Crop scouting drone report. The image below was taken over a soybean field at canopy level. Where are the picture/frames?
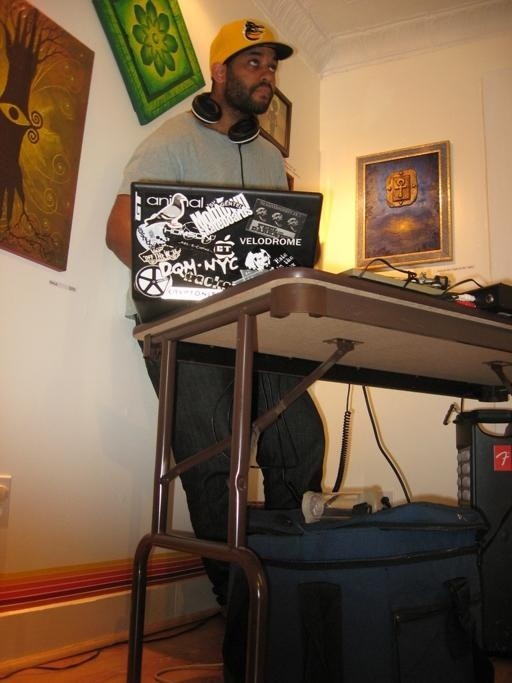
[261,84,292,157]
[354,141,453,270]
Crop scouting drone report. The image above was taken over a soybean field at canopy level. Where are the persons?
[106,18,326,605]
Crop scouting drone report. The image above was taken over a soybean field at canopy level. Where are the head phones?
[191,92,261,144]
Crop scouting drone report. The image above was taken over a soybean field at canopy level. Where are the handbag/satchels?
[221,489,494,683]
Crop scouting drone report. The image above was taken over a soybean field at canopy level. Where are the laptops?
[128,181,324,323]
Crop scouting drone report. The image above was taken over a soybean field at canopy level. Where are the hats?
[210,18,294,77]
